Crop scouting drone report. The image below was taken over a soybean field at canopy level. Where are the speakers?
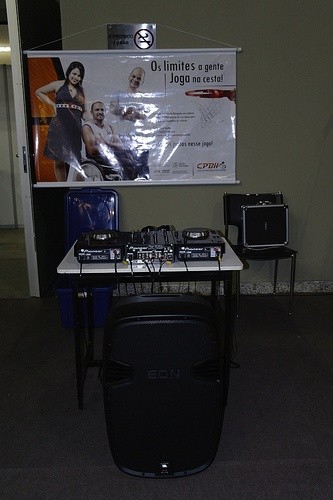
[101,293,230,479]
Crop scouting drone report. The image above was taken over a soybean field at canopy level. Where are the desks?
[57,237,243,411]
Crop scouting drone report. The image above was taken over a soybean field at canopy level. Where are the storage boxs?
[54,275,112,328]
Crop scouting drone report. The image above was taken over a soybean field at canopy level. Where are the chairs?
[223,191,299,318]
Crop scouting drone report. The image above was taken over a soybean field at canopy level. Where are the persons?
[34,61,85,182]
[108,67,151,180]
[81,101,150,181]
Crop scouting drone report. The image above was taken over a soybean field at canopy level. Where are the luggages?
[98,293,231,479]
